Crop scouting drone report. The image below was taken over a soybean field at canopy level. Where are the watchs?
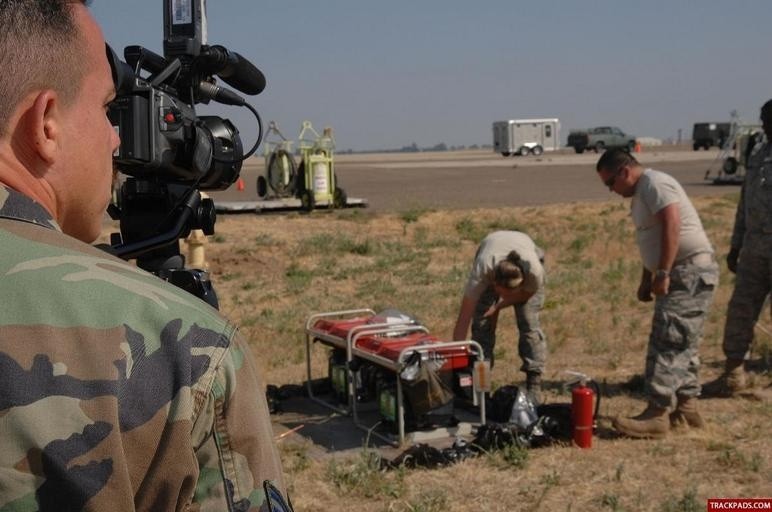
[656,269,670,279]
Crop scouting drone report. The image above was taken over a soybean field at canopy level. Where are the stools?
[302,307,486,447]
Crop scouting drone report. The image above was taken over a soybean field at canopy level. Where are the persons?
[699,99,772,396]
[0,0,292,511]
[452,230,548,409]
[595,147,719,439]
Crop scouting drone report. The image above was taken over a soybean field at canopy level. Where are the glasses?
[605,161,629,186]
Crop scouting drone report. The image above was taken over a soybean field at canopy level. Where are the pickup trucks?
[566,127,636,155]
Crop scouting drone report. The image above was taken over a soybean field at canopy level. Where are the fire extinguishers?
[567,371,601,449]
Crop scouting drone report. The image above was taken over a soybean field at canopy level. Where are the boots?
[670,395,701,431]
[613,407,671,438]
[701,359,746,399]
[526,371,542,411]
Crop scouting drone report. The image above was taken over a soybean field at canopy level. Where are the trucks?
[692,122,729,150]
